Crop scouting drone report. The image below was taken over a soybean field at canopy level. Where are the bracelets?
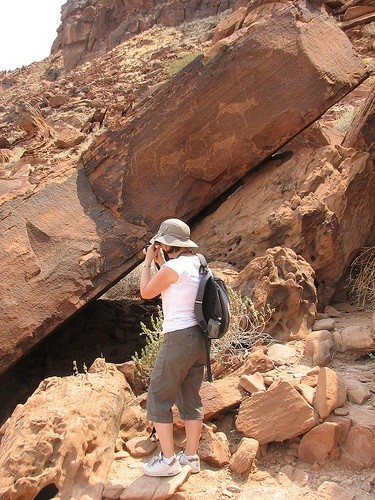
[142,264,151,268]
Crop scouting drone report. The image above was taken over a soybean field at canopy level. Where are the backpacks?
[195,254,231,339]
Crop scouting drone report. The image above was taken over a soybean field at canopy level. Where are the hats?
[149,218,199,247]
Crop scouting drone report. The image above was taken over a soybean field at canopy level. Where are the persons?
[139,218,214,475]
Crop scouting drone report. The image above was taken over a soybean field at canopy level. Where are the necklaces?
[176,251,192,258]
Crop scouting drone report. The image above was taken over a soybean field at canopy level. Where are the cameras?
[143,245,160,256]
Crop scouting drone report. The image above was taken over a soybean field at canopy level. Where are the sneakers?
[142,450,181,477]
[177,451,200,473]
